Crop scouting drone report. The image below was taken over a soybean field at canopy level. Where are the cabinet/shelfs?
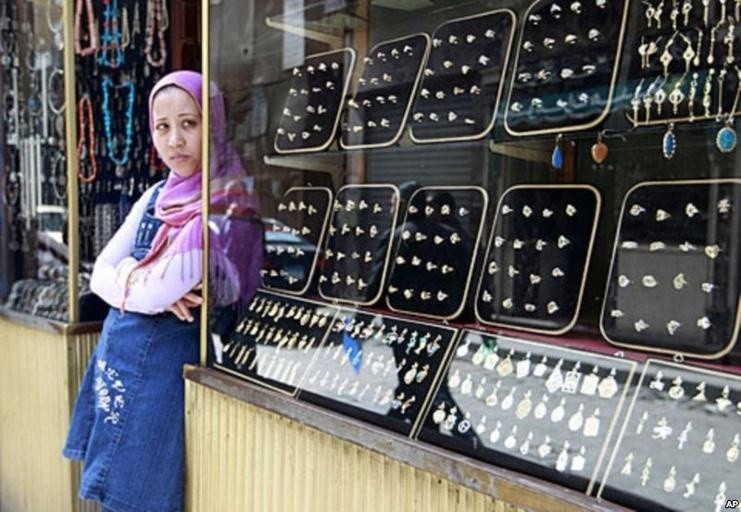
[1,2,177,512]
[183,0,738,512]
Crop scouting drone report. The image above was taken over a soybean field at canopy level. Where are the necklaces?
[225,297,739,512]
[1,0,169,324]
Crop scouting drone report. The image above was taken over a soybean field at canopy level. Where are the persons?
[65,70,264,512]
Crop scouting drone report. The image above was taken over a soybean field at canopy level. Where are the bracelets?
[630,0,740,128]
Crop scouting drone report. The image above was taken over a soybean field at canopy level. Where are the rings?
[276,0,607,141]
[261,197,732,339]
